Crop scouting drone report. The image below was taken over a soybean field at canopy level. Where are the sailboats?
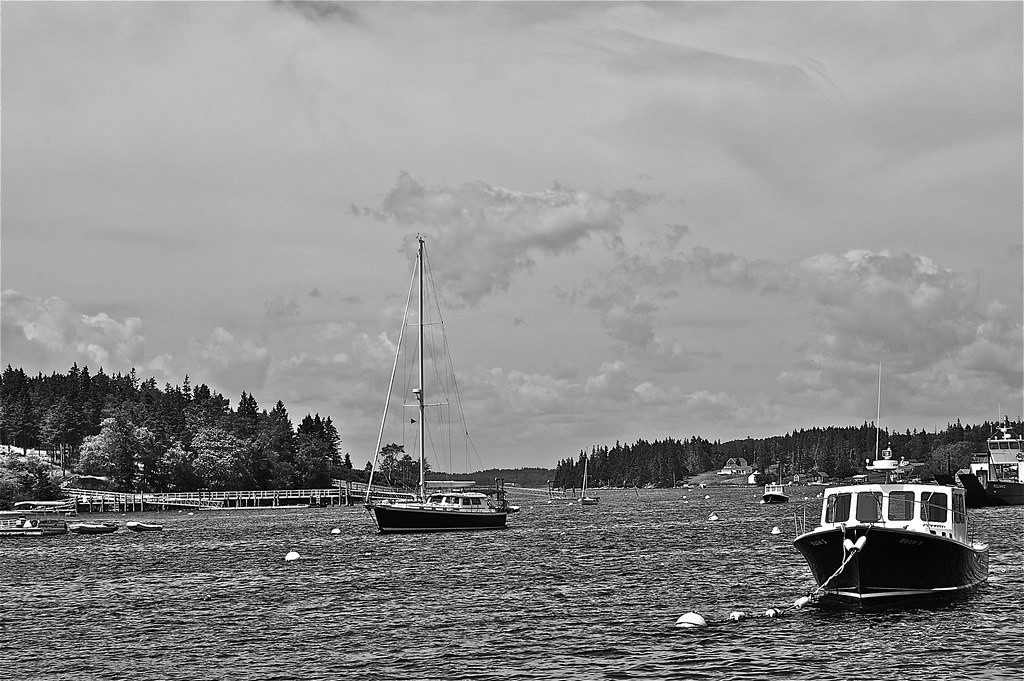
[578,458,601,506]
[362,229,510,535]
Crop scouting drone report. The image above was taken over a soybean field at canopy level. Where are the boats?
[791,358,997,609]
[952,403,1024,511]
[0,511,71,538]
[68,519,120,535]
[762,461,793,504]
[126,521,163,533]
